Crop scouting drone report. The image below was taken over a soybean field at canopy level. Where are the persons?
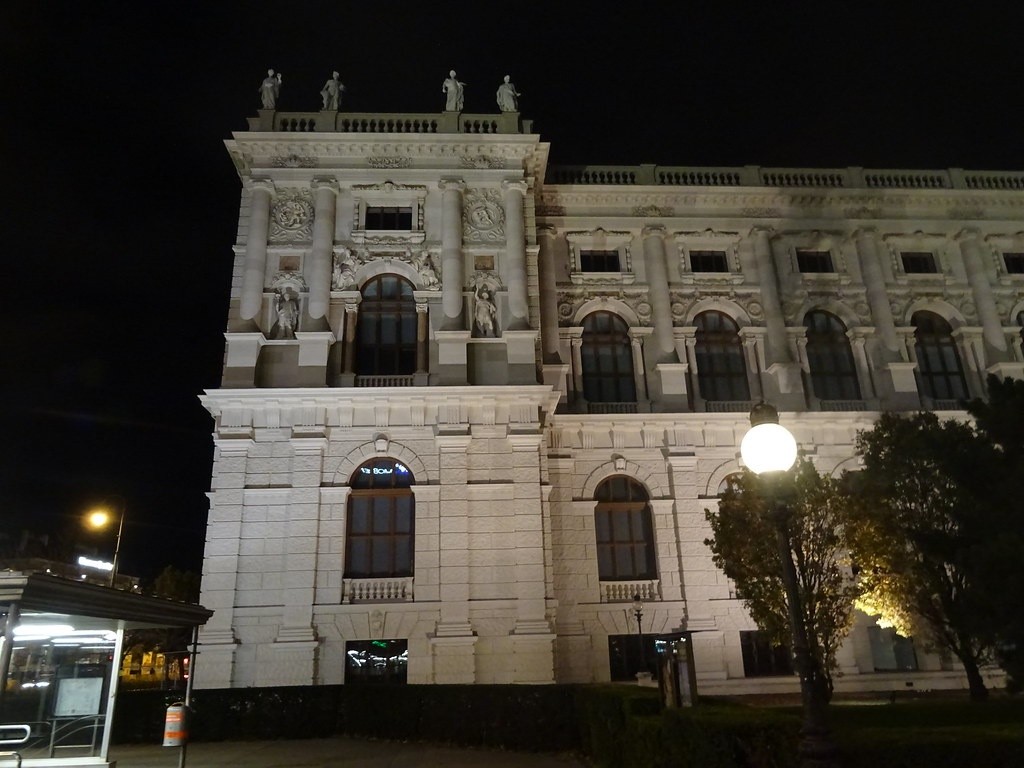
[258,70,282,109]
[497,75,521,112]
[474,284,496,338]
[275,293,300,338]
[320,71,344,110]
[443,70,466,112]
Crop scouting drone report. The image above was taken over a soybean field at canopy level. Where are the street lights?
[92,494,128,588]
[741,400,839,768]
[632,594,654,685]
